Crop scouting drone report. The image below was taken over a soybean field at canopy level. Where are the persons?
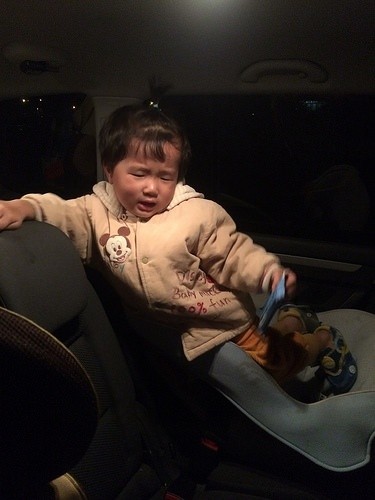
[0,98,359,394]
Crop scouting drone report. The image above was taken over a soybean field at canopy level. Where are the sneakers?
[277,303,321,332]
[314,325,357,393]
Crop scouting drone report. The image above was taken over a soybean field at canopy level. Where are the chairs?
[0,219,375,500]
